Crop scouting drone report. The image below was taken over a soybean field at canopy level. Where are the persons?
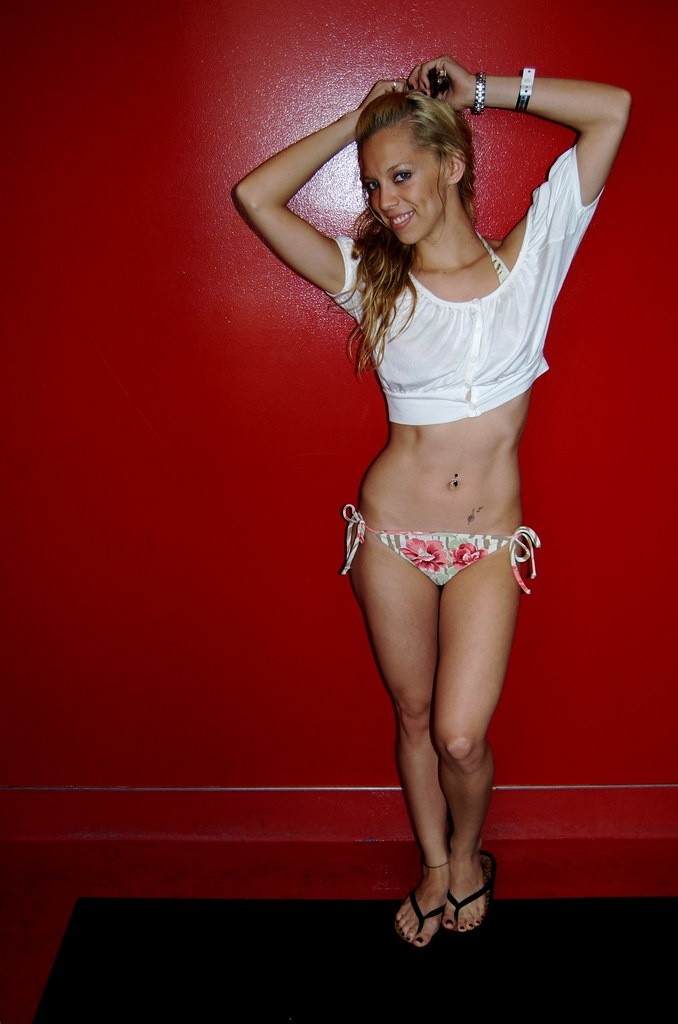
[232,52,633,950]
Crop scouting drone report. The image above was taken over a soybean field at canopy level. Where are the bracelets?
[471,71,487,117]
[513,67,536,113]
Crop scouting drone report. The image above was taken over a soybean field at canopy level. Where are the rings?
[435,70,447,78]
[392,80,399,91]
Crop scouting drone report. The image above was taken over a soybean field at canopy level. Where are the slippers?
[440,849,497,938]
[393,885,445,954]
